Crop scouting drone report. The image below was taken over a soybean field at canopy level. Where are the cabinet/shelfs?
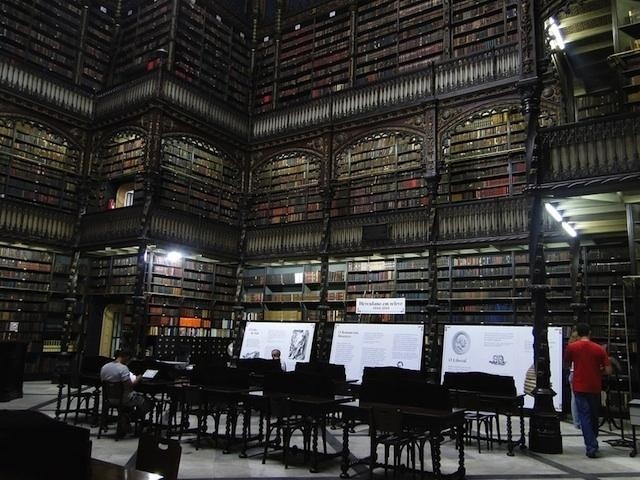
[1,1,639,344]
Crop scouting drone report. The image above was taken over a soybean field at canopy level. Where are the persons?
[289,331,307,360]
[100,348,147,435]
[271,349,288,372]
[567,328,582,429]
[563,321,613,459]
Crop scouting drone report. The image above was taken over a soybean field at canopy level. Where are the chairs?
[48,350,527,478]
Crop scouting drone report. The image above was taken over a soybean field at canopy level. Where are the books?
[587,329,640,413]
[129,372,137,383]
[1,0,639,328]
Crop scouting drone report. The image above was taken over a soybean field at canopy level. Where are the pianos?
[441,372,525,456]
[336,366,468,477]
[54,354,117,433]
[175,353,355,473]
[123,359,193,441]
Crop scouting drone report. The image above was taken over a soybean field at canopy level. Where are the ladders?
[608,284,632,407]
